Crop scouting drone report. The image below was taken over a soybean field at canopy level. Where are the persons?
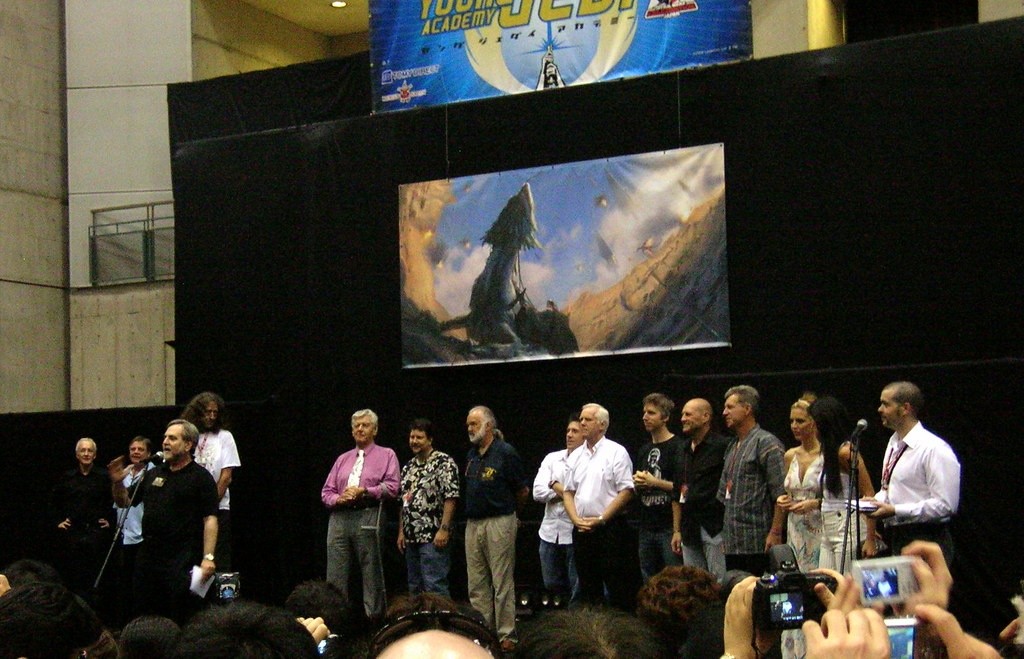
[782,394,888,574]
[0,541,1024,659]
[322,409,401,616]
[181,392,241,604]
[463,406,529,651]
[716,385,785,595]
[633,392,731,589]
[397,419,461,598]
[107,419,220,626]
[532,402,636,615]
[43,435,157,636]
[861,381,962,565]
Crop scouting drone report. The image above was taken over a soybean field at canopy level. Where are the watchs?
[599,515,607,525]
[440,523,450,531]
[549,479,559,489]
[203,553,215,561]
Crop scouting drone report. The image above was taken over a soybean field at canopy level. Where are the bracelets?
[817,497,823,507]
[363,486,368,494]
[673,529,681,533]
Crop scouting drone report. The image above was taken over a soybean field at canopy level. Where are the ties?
[882,445,906,505]
[347,450,365,489]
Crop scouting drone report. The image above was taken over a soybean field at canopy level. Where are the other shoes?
[500,640,514,652]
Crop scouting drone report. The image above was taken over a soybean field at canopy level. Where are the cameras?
[881,614,940,659]
[317,634,339,655]
[751,560,838,632]
[848,556,922,605]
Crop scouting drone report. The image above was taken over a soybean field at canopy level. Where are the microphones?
[139,450,164,463]
[850,419,869,440]
